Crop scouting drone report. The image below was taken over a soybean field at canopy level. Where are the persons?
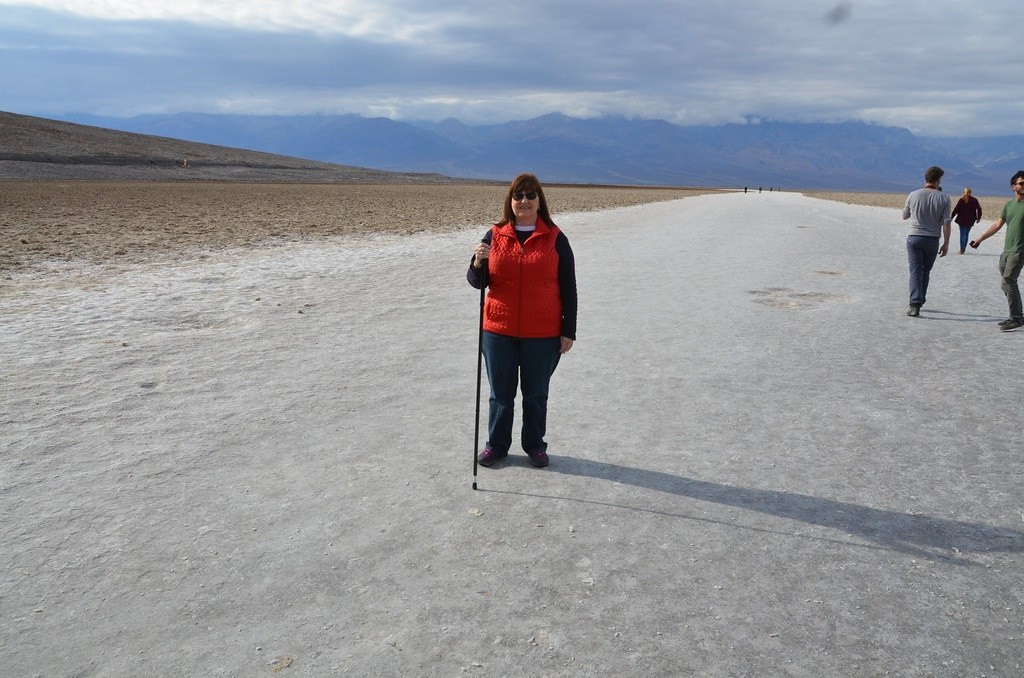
[902,166,951,315]
[466,173,578,467]
[970,171,1024,331]
[951,189,982,254]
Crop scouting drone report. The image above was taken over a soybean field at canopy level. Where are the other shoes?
[906,306,919,316]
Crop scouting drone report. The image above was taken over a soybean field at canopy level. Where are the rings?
[481,251,483,254]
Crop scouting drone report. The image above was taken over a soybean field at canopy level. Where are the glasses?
[511,192,537,200]
[1015,182,1024,186]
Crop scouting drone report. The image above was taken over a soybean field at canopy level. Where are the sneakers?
[477,447,509,466]
[521,444,550,467]
[999,319,1023,331]
[999,316,1024,325]
